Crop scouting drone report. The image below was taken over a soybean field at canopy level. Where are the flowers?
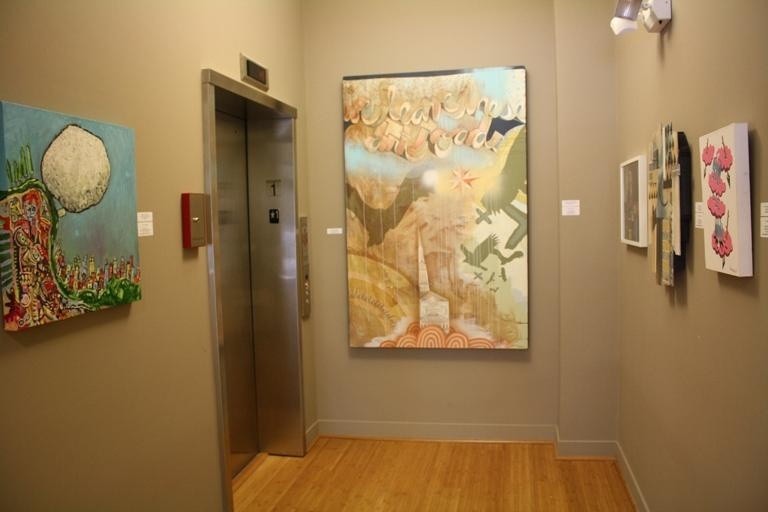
[702,136,733,269]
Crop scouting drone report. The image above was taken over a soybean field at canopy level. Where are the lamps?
[610,0,672,35]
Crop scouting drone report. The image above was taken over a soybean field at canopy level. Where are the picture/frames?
[620,155,648,247]
